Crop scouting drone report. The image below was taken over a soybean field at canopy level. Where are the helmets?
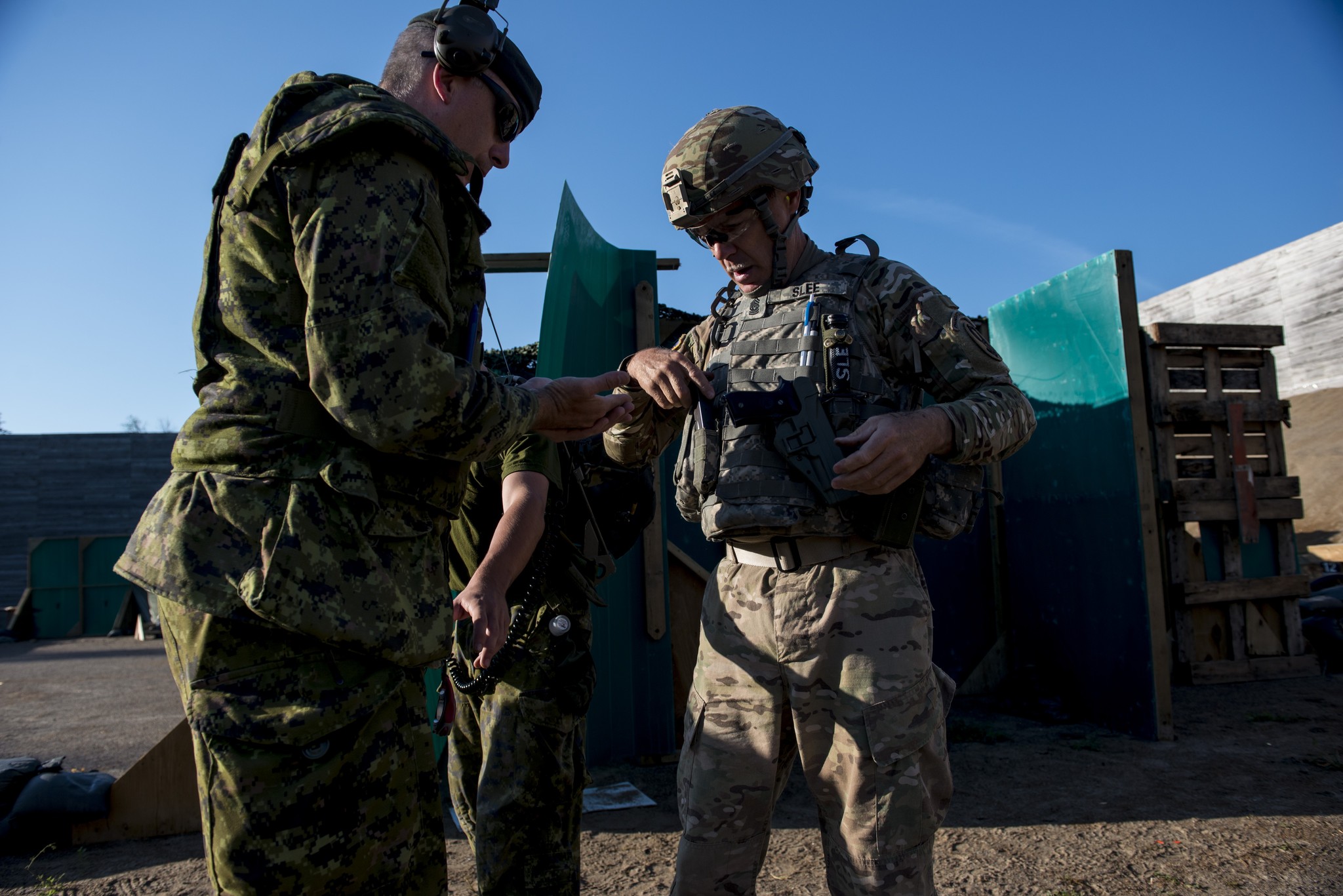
[659,105,819,231]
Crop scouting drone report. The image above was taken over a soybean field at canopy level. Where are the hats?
[406,7,543,135]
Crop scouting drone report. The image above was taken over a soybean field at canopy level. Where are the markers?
[806,302,821,365]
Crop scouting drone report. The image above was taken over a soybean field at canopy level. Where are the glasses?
[420,51,521,143]
[685,201,760,249]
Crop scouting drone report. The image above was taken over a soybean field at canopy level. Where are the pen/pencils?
[800,294,814,366]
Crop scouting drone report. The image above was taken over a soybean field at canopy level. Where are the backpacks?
[557,433,657,562]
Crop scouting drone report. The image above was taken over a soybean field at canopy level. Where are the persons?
[113,1,633,896]
[443,339,600,896]
[602,104,1039,896]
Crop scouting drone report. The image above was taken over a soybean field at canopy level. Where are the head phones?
[433,2,508,77]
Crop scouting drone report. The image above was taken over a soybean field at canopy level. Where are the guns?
[720,375,857,506]
